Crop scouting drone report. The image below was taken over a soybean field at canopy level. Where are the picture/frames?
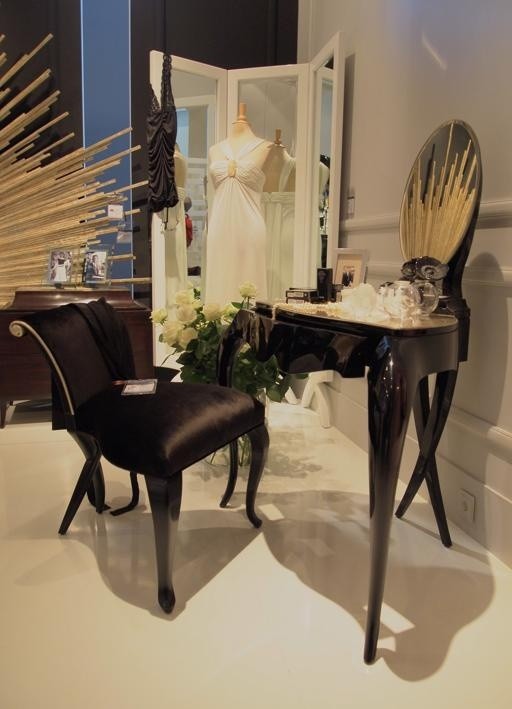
[0,287,155,429]
[217,300,472,667]
[332,248,368,290]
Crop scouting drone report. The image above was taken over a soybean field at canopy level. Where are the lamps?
[8,296,271,615]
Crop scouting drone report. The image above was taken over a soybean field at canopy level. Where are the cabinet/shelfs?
[375,286,390,314]
[383,281,421,320]
[407,278,439,317]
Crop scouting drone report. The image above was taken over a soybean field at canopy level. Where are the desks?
[217,300,472,667]
[0,287,155,429]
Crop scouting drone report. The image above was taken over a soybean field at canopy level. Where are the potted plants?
[332,248,368,290]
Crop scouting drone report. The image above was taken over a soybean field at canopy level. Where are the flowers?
[148,281,308,467]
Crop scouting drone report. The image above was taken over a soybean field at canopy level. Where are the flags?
[204,418,272,480]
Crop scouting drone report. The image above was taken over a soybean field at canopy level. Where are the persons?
[50,251,71,284]
[342,267,353,288]
[165,103,331,342]
[87,255,104,280]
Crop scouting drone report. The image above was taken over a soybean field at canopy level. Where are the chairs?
[8,296,271,615]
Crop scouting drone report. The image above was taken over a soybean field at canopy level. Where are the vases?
[148,281,308,467]
[204,418,272,480]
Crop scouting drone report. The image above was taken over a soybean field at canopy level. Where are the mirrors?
[148,50,226,310]
[309,32,345,290]
[398,119,484,266]
[227,63,309,299]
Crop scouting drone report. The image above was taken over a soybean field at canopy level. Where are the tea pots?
[398,119,484,266]
